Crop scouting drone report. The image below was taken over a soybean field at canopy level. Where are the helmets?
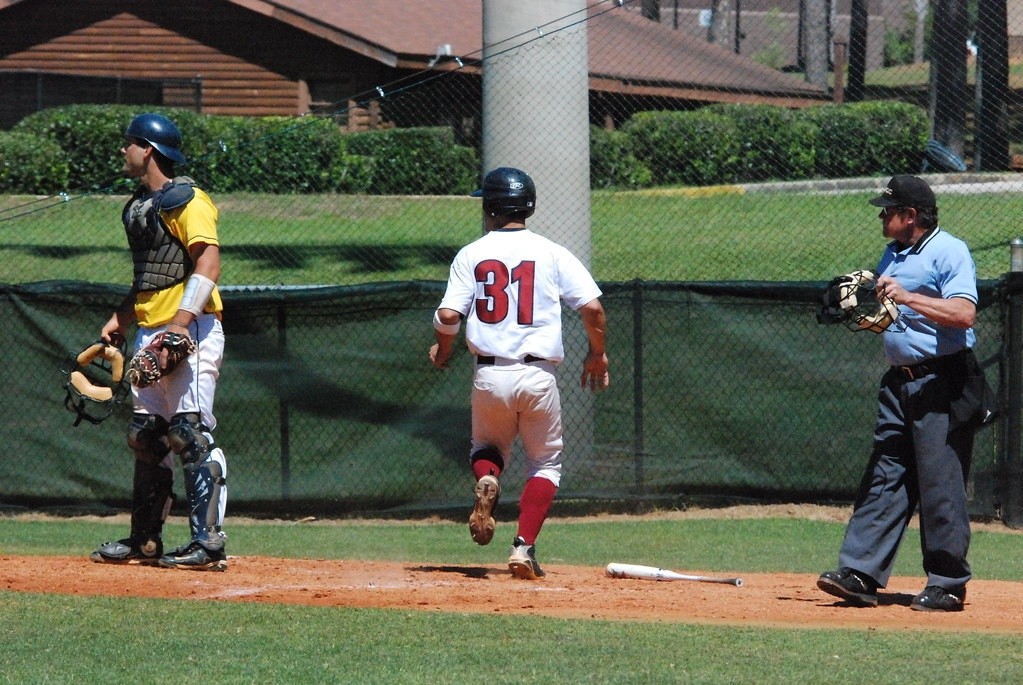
[471,168,536,217]
[126,113,186,162]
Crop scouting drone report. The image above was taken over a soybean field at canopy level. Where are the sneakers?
[911,586,967,612]
[159,541,227,570]
[469,468,501,545]
[90,537,163,565]
[818,568,878,606]
[510,536,546,579]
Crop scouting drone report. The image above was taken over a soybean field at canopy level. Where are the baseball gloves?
[129,331,196,388]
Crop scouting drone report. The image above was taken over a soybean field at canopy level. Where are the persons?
[97,113,228,566]
[817,176,978,610]
[428,166,609,580]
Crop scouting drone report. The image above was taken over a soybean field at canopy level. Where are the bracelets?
[432,311,461,335]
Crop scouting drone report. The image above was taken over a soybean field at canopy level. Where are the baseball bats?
[605,562,743,587]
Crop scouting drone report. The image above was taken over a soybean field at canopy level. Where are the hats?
[869,175,935,207]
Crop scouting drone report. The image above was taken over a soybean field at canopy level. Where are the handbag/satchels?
[935,350,1000,444]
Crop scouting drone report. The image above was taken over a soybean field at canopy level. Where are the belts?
[478,354,546,364]
[894,357,941,381]
